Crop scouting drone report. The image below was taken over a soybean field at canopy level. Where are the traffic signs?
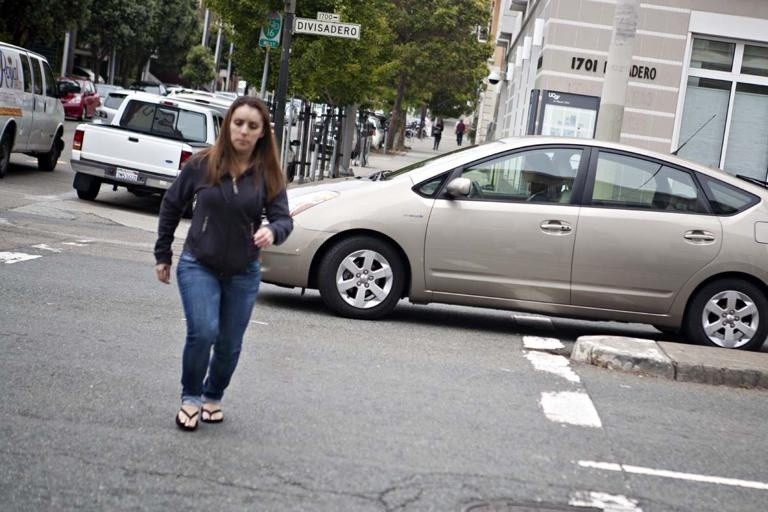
[293,10,362,41]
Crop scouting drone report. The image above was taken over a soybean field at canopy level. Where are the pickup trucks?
[71,92,223,219]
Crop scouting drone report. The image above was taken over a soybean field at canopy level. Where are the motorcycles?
[406,121,426,138]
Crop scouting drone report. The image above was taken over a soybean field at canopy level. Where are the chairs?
[556,171,576,202]
[650,184,670,209]
[666,188,695,211]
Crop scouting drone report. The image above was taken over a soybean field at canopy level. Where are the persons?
[432,116,443,150]
[456,119,465,146]
[521,153,560,201]
[154,96,293,430]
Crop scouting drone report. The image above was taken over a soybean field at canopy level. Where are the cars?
[259,134,764,350]
[89,88,144,127]
[56,75,101,121]
[146,81,386,178]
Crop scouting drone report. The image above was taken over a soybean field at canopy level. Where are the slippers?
[176,406,200,432]
[201,407,223,424]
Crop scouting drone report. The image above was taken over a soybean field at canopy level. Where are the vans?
[0,40,68,178]
